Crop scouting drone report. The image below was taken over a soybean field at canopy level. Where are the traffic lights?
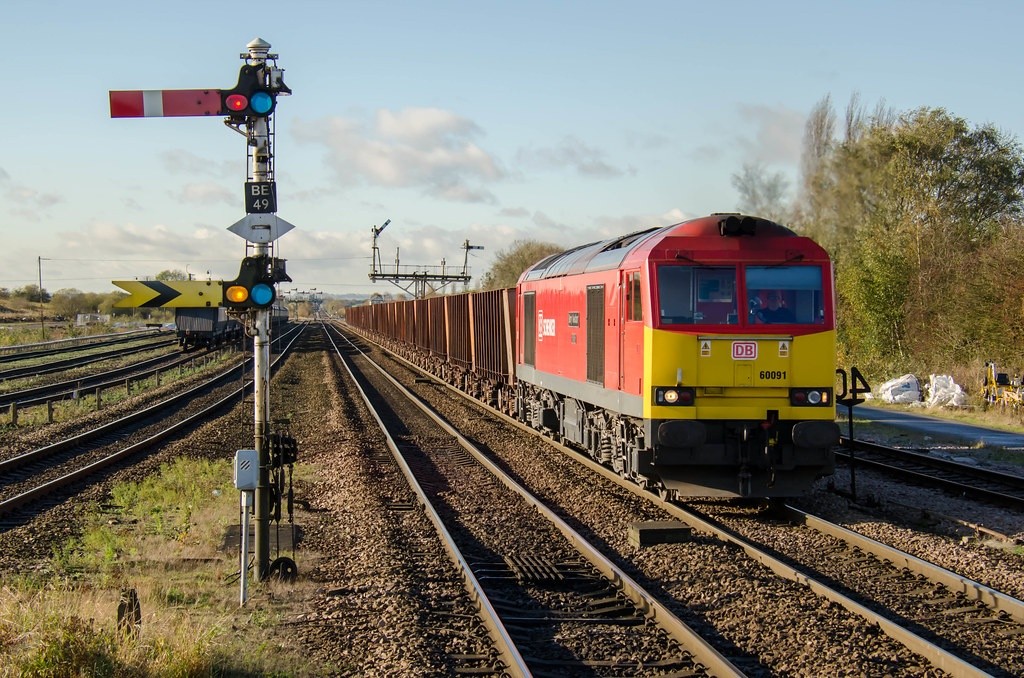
[218,94,277,115]
[225,280,276,308]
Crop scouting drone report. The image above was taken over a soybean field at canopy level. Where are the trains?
[342,215,845,498]
